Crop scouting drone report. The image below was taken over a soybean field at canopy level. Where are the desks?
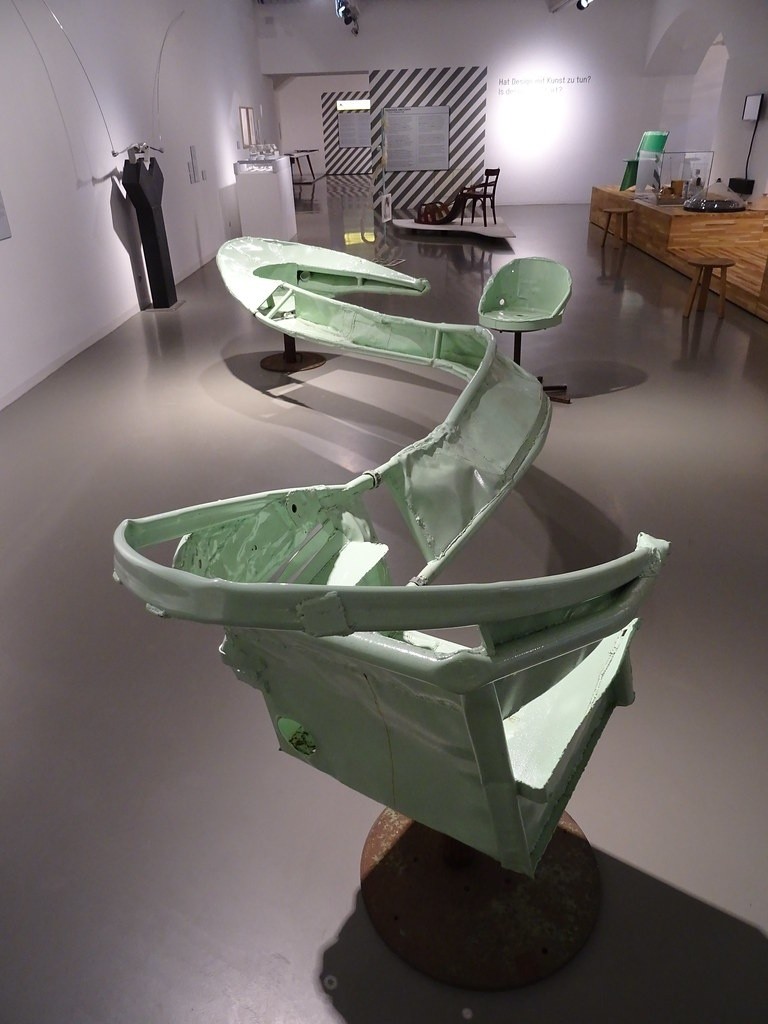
[682,256,736,319]
[287,147,319,180]
[601,207,633,251]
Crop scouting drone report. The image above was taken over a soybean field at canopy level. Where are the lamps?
[335,0,359,38]
[336,99,370,110]
[729,91,765,194]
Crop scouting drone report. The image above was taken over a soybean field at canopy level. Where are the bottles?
[691,168,703,186]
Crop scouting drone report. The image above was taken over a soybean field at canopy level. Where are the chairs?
[417,167,503,228]
[125,232,664,976]
